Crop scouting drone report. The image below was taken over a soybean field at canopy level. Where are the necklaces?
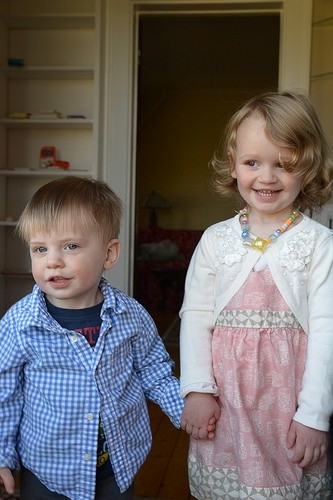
[238,204,301,251]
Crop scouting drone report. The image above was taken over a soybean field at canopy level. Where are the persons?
[176,92,333,500]
[1,174,216,500]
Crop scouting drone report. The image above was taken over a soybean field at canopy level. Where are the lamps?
[138,190,175,226]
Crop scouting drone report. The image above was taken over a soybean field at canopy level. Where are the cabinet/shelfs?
[0,0,105,318]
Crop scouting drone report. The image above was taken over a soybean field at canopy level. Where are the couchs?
[134,228,207,314]
